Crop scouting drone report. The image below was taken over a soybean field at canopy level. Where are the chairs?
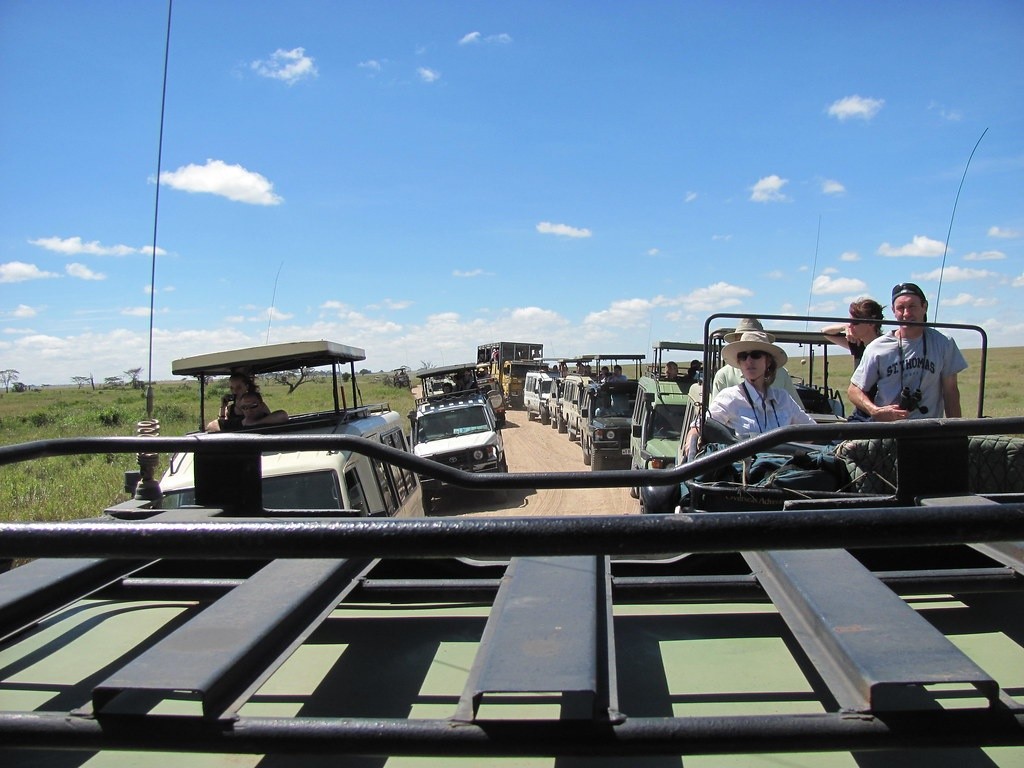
[427,416,445,438]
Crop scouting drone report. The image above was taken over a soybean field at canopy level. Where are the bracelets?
[218,415,226,418]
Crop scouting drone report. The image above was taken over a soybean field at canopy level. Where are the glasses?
[852,320,870,325]
[240,402,261,410]
[737,350,766,361]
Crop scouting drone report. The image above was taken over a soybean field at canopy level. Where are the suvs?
[407,362,508,514]
[630,339,848,507]
[392,368,413,392]
[675,312,1023,509]
[417,342,648,471]
[157,338,427,519]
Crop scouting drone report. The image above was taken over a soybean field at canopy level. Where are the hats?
[892,283,927,323]
[724,318,775,343]
[721,331,788,369]
[691,360,702,368]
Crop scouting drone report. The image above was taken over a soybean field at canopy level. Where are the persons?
[442,367,491,393]
[491,348,541,361]
[681,332,818,460]
[400,369,405,375]
[819,300,886,422]
[207,393,289,430]
[576,362,591,376]
[848,282,968,422]
[217,374,260,419]
[552,362,572,378]
[664,360,704,382]
[599,365,626,383]
[712,318,806,413]
